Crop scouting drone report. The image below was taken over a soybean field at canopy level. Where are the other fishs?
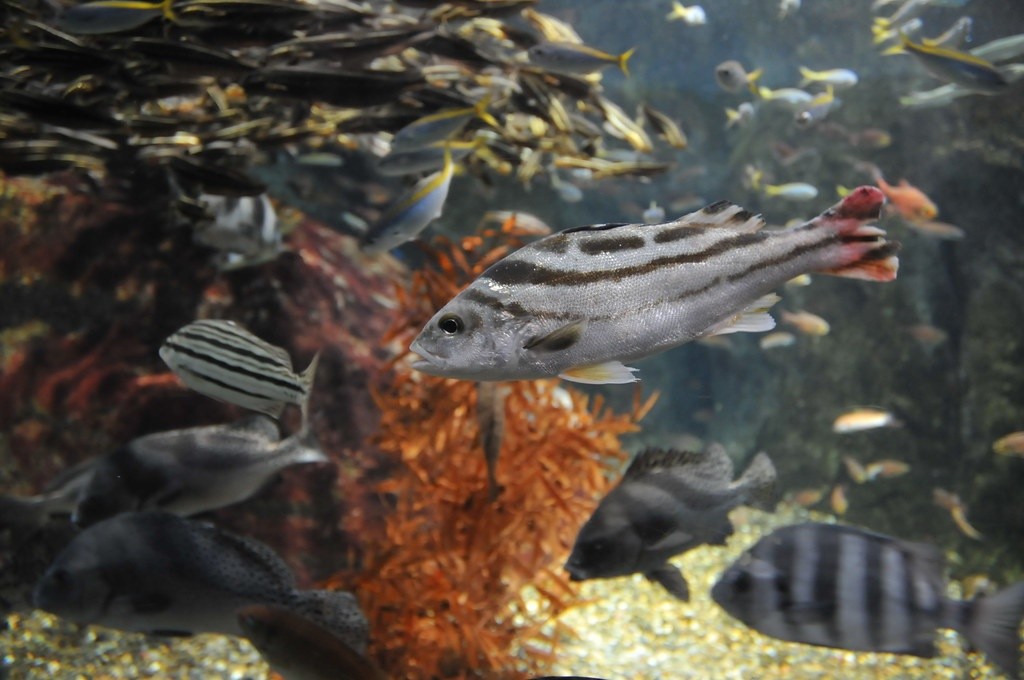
[0,0,1024,680]
